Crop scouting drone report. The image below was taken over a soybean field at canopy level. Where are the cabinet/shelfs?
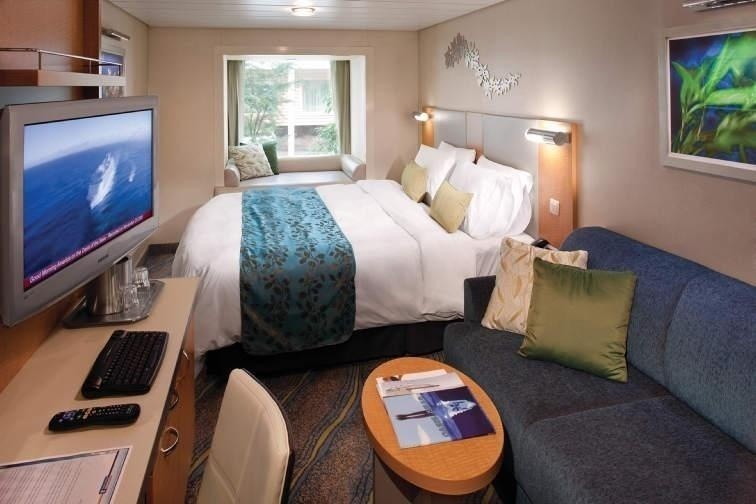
[139,298,196,503]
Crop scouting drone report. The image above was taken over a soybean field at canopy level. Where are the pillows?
[227,140,279,182]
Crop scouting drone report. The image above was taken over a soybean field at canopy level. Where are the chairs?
[197,365,296,503]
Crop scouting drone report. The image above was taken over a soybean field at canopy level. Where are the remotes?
[49,403,140,430]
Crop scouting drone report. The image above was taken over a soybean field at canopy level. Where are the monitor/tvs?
[0,95,163,328]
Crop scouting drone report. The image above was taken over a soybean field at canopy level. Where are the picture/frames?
[656,12,756,184]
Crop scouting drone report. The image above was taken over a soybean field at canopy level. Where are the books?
[374,368,497,449]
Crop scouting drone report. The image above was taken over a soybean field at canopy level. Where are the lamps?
[523,127,570,146]
[410,110,434,123]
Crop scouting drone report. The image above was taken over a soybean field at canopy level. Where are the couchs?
[440,225,756,503]
[213,152,366,195]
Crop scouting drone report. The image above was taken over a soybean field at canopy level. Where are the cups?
[84,255,137,316]
[131,266,150,290]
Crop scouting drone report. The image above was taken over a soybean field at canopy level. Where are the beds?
[183,104,579,379]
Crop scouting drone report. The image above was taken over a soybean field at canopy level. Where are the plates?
[63,278,167,328]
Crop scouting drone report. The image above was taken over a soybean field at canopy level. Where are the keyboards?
[81,329,169,400]
